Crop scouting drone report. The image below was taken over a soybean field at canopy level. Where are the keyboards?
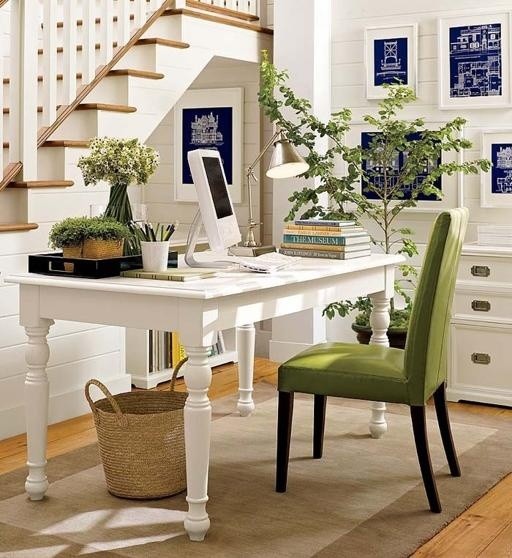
[240,256,297,273]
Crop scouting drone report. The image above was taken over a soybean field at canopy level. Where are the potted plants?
[251,46,493,350]
[49,216,127,261]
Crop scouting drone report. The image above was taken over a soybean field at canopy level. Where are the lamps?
[230,121,311,257]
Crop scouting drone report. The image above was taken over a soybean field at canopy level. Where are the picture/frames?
[172,84,247,208]
[335,8,512,219]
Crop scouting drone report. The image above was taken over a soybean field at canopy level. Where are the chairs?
[272,206,464,510]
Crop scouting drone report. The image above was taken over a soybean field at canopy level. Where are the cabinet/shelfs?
[123,237,239,389]
[444,241,511,409]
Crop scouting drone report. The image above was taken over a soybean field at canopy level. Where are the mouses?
[259,252,286,258]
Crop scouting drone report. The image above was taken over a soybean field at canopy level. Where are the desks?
[1,248,404,541]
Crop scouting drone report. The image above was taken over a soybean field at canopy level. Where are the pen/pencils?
[128,220,179,241]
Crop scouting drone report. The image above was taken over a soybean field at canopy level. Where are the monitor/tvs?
[184,149,242,269]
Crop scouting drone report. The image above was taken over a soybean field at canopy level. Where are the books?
[278,219,371,260]
[123,268,219,281]
[149,329,226,373]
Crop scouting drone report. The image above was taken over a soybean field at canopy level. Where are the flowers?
[76,134,160,223]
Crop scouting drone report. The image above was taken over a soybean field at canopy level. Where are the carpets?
[0,381,511,558]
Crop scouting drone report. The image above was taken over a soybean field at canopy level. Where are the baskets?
[62,236,125,259]
[85,355,189,500]
